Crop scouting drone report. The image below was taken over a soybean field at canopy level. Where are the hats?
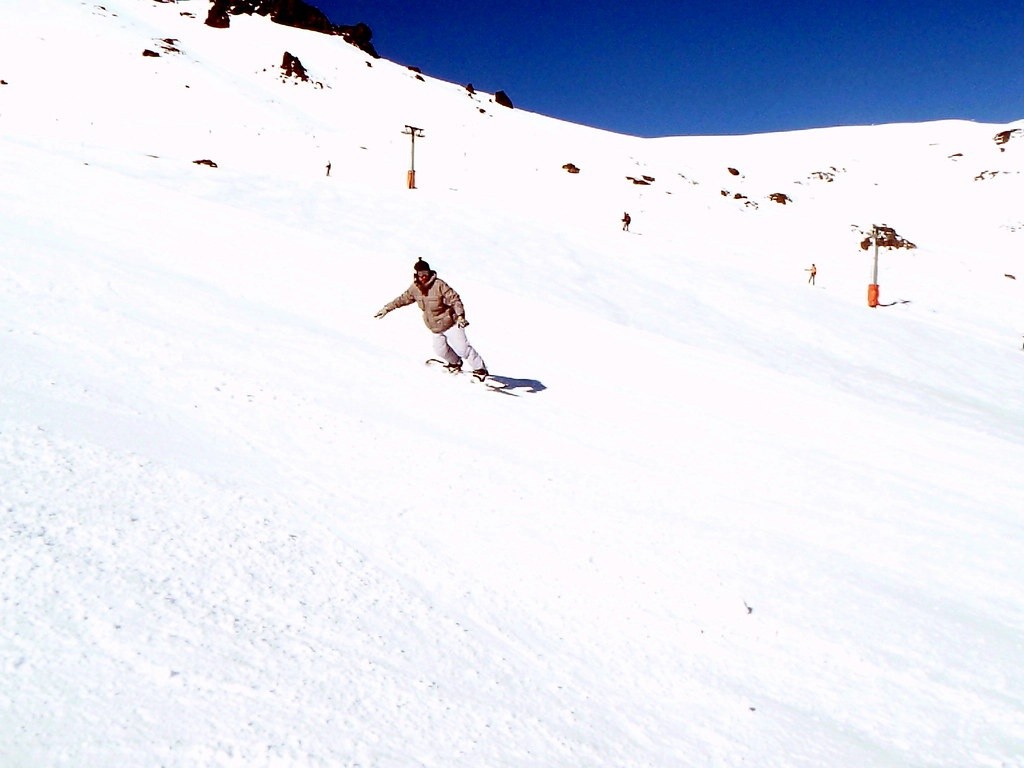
[415,257,430,271]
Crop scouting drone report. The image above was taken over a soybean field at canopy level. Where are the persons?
[326,160,332,176]
[622,214,631,231]
[374,260,489,383]
[809,264,816,285]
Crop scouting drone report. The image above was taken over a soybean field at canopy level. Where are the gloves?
[457,316,469,328]
[374,307,388,319]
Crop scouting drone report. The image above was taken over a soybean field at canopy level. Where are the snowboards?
[426,358,509,391]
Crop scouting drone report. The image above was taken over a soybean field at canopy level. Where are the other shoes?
[443,360,463,371]
[473,369,489,375]
